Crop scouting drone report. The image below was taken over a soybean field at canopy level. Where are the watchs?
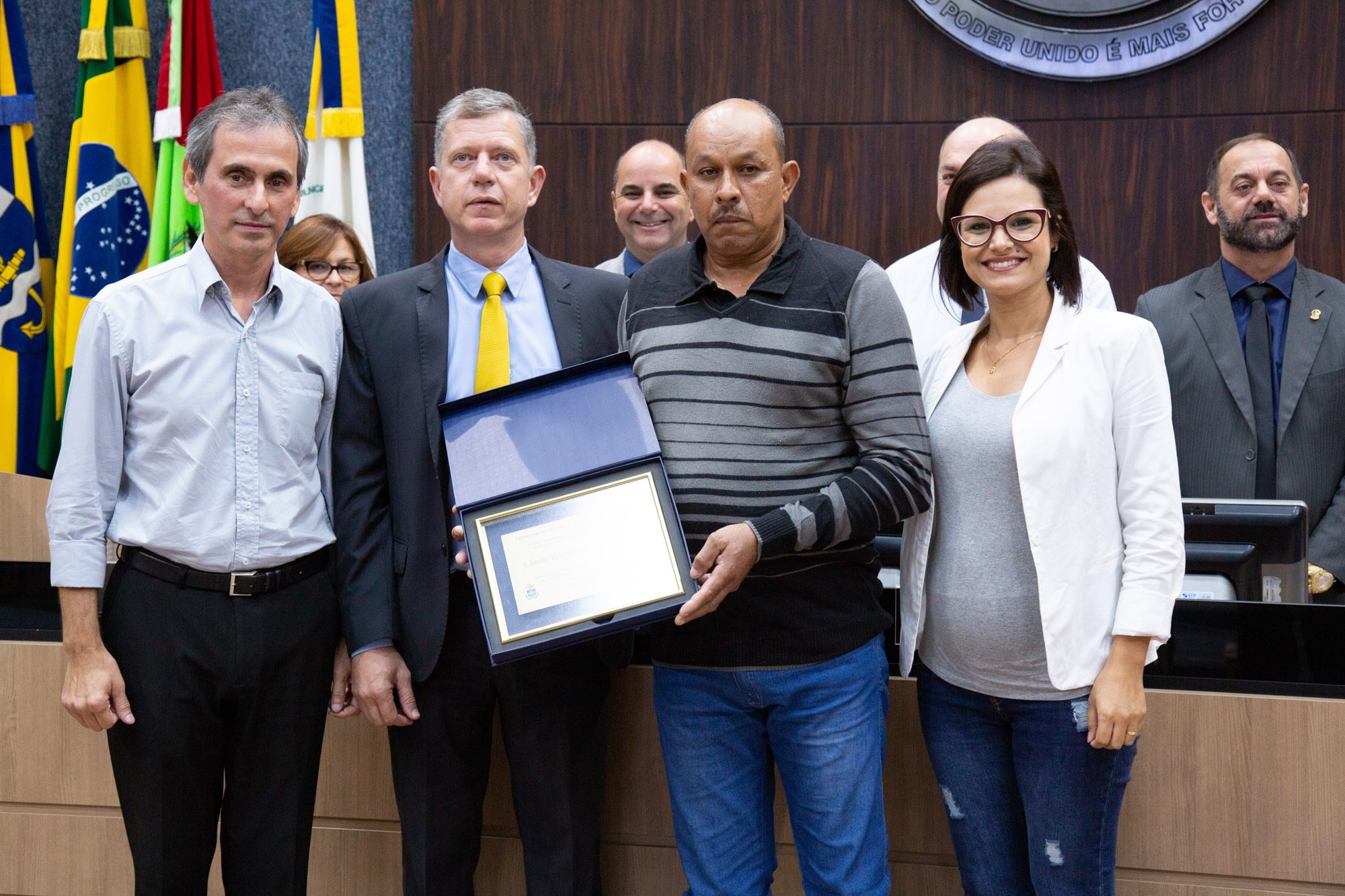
[1307,562,1335,595]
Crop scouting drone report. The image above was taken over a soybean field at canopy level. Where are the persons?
[330,87,632,896]
[883,116,1118,415]
[452,98,932,896]
[594,139,697,276]
[45,81,362,896]
[277,212,377,316]
[899,139,1187,896]
[1132,133,1344,610]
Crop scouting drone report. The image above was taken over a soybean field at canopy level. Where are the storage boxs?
[441,355,699,667]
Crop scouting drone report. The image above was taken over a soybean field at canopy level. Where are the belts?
[121,546,336,596]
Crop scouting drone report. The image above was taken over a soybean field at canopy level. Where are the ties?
[1242,284,1280,499]
[960,287,985,324]
[473,272,510,394]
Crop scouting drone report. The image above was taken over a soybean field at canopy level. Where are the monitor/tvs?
[1177,497,1308,604]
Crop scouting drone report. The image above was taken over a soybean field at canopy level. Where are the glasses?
[950,208,1052,247]
[294,259,364,282]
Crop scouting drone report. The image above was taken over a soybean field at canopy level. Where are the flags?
[0,1,365,479]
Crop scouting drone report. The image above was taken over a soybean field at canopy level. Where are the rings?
[1126,731,1137,737]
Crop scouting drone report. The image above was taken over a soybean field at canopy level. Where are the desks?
[2,601,1345,894]
